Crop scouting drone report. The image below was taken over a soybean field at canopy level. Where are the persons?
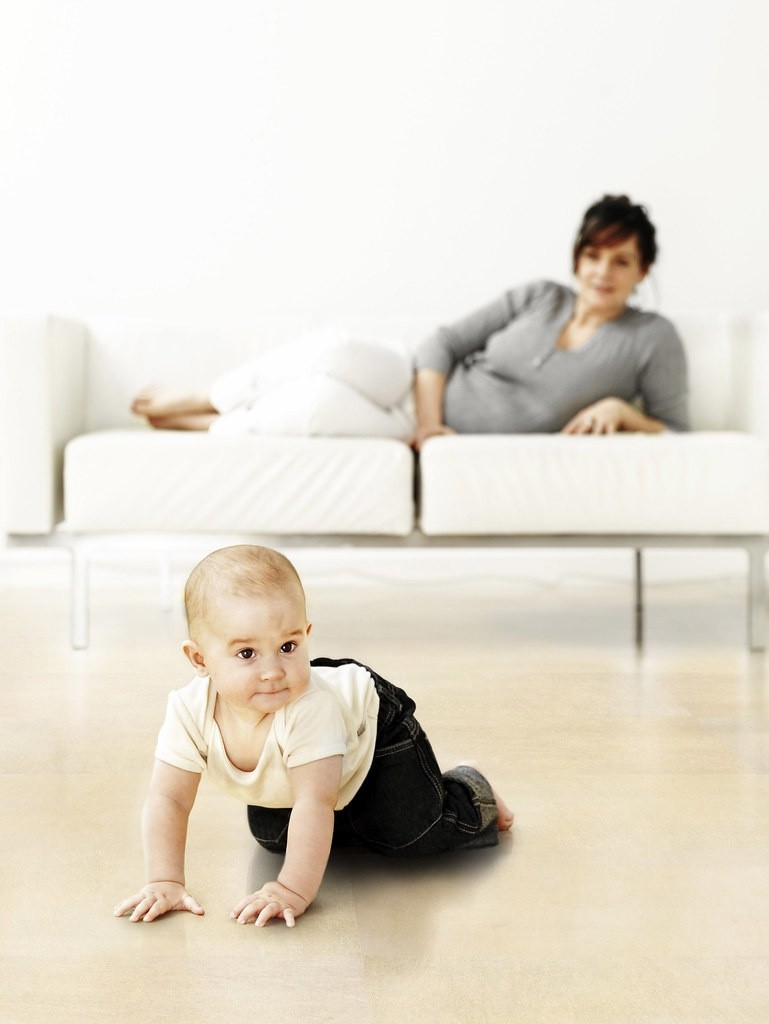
[127,196,691,441]
[113,544,514,929]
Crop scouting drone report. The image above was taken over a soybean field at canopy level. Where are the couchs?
[0,309,769,654]
